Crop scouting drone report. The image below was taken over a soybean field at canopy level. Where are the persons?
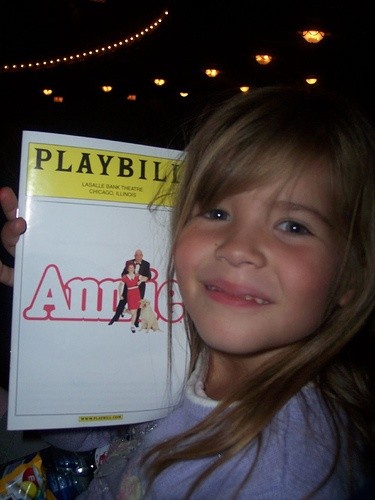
[118,261,148,332]
[108,249,151,328]
[0,83,375,500]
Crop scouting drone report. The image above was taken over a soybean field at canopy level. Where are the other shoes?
[131,327,135,334]
[134,322,139,328]
[108,318,119,326]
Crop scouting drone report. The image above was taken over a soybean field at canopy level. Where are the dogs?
[137,299,163,333]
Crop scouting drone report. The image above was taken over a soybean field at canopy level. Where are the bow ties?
[135,262,142,267]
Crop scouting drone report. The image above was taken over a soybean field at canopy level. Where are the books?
[6,131,190,431]
